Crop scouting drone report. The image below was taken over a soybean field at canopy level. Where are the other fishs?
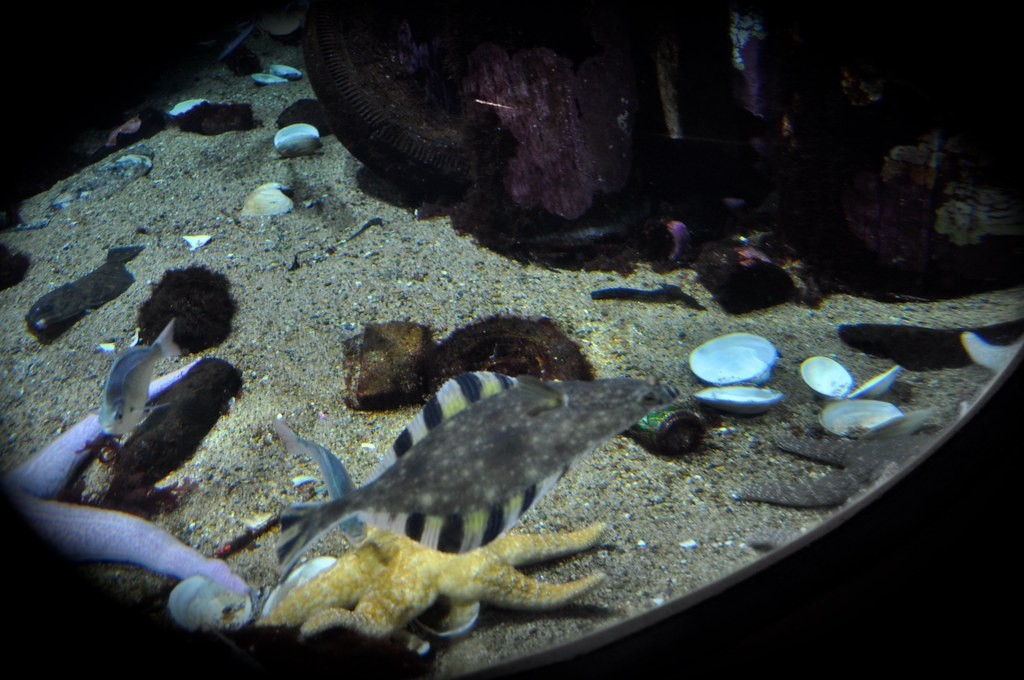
[25,246,146,346]
[96,317,181,435]
[255,371,680,588]
